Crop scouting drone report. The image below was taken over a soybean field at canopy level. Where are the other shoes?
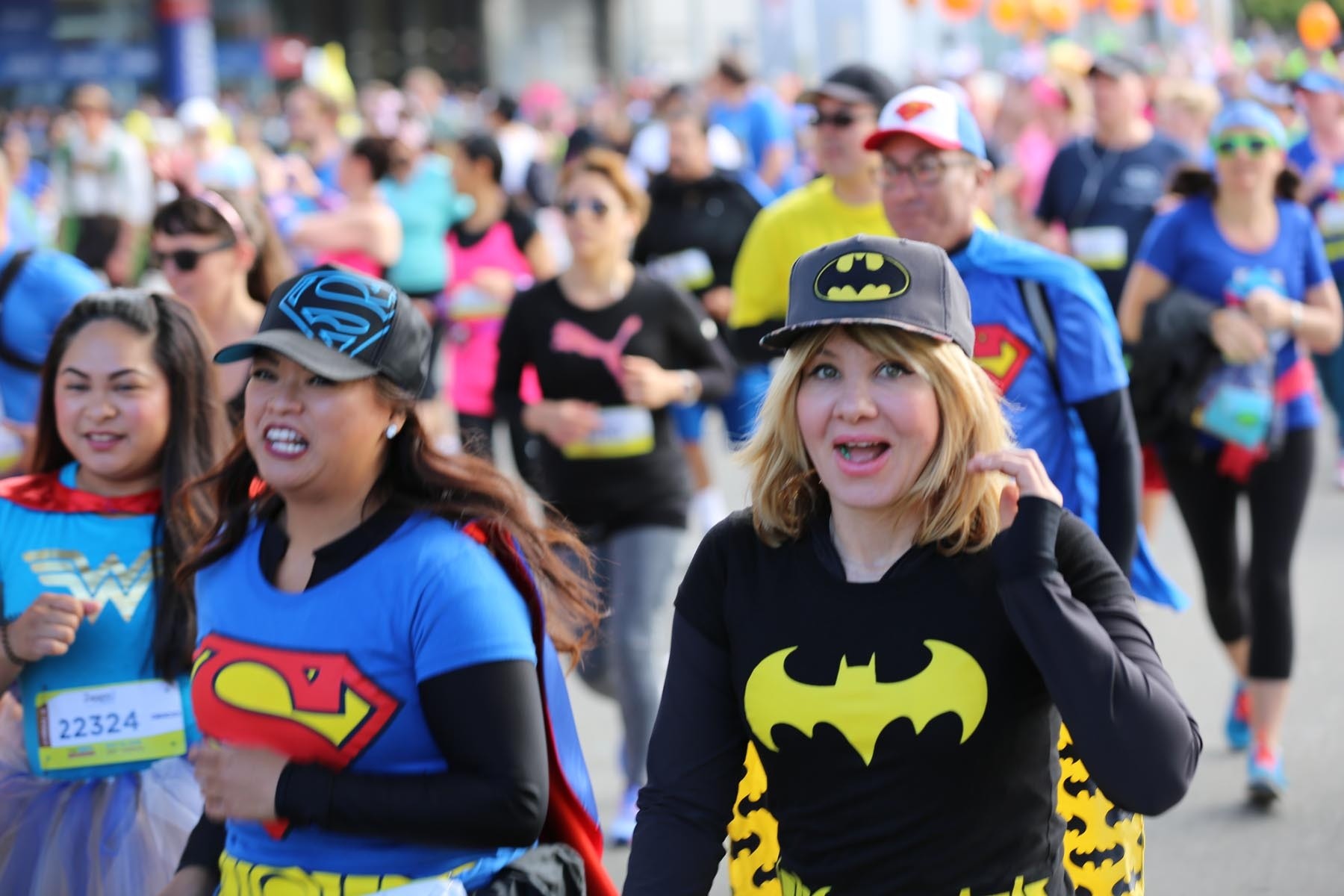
[610,782,642,845]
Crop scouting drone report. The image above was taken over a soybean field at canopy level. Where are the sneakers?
[1247,740,1281,799]
[1224,684,1251,752]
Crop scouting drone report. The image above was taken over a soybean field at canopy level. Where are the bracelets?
[2,623,26,665]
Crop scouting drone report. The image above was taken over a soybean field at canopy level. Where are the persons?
[491,145,738,846]
[621,237,1203,896]
[158,262,616,896]
[797,82,1168,601]
[1115,99,1343,811]
[1,32,1343,562]
[0,288,231,896]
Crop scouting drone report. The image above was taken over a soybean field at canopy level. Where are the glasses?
[1214,134,1278,158]
[556,198,624,218]
[807,108,874,131]
[149,244,230,273]
[870,150,974,189]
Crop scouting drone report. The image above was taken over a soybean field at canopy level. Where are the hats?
[1208,101,1292,154]
[1085,51,1151,82]
[795,65,898,108]
[214,262,431,400]
[864,84,989,163]
[758,235,975,360]
[1292,71,1344,95]
